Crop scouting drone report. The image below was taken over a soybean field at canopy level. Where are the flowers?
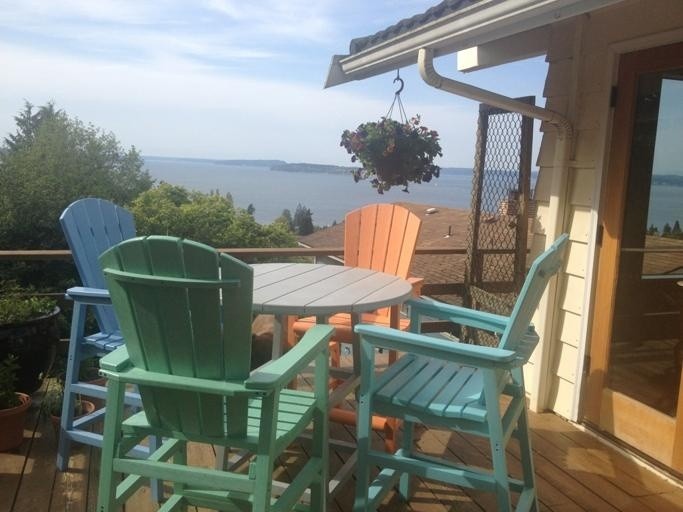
[334,115,444,197]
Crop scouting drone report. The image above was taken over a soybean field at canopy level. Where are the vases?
[375,157,405,184]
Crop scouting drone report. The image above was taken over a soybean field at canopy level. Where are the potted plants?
[0,278,107,455]
